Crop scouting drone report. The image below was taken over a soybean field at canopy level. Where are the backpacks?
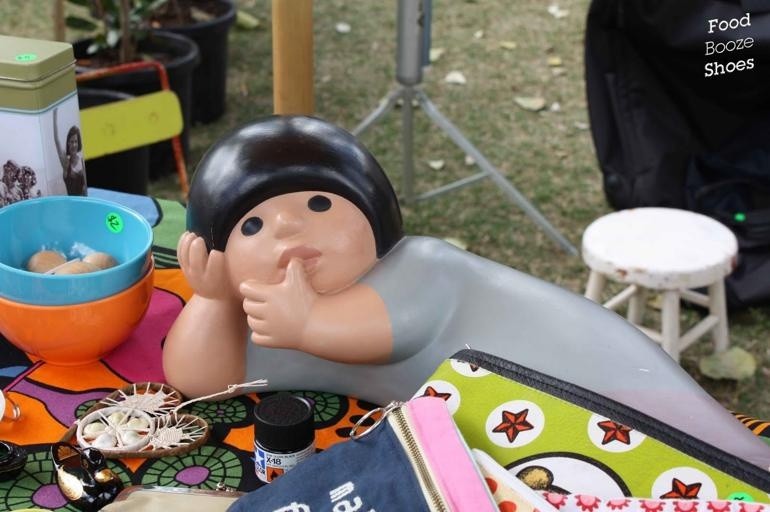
[583,0,770,317]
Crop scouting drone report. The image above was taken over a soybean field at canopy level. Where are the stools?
[578,205,744,364]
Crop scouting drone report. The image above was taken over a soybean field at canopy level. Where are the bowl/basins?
[0,193,156,370]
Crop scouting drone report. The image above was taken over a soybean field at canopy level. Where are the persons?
[52,106,88,196]
[161,115,769,473]
